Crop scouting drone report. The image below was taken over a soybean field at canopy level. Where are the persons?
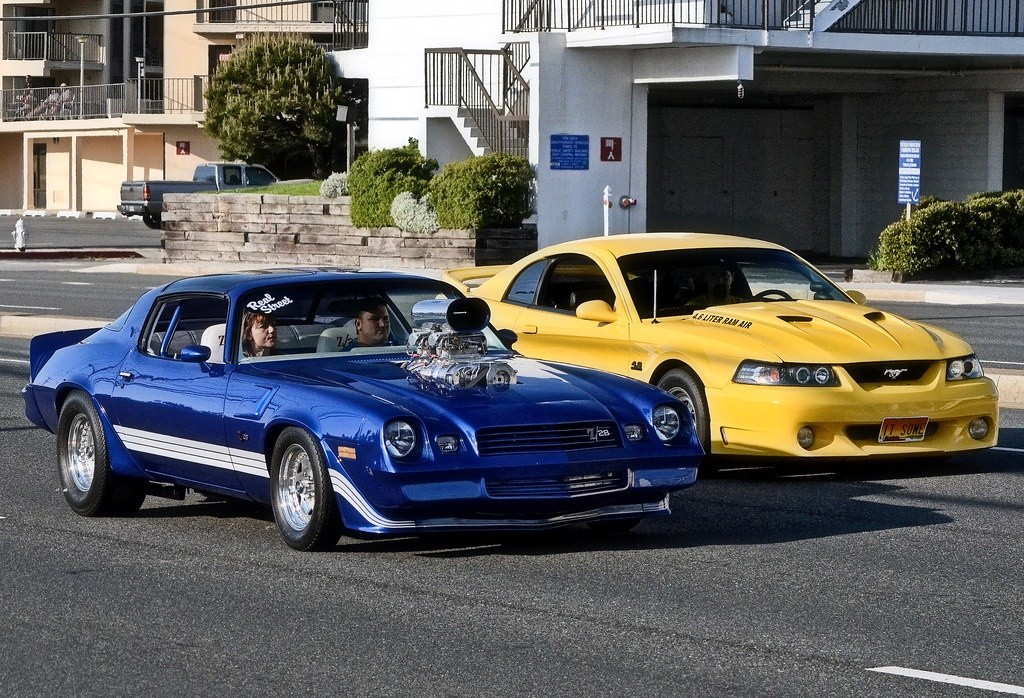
[689,267,752,304]
[16,74,74,119]
[345,294,398,346]
[239,312,283,358]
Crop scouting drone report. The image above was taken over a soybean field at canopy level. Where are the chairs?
[2,94,76,120]
[571,275,696,310]
[316,326,358,352]
[202,323,227,361]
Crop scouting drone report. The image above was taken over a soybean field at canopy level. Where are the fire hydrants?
[11,218,30,252]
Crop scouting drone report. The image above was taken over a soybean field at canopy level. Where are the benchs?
[150,323,340,355]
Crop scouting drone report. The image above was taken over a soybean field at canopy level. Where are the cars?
[435,232,1000,478]
[21,266,707,552]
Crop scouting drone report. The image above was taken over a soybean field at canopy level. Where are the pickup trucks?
[117,163,314,229]
